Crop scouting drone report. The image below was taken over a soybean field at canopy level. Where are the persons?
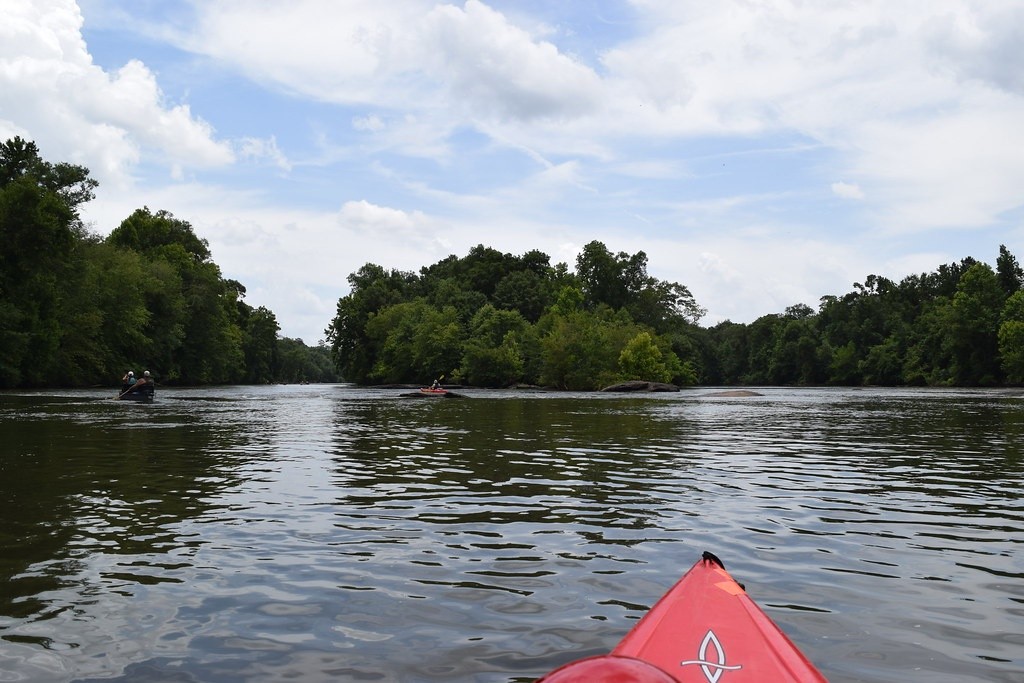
[123,370,153,387]
[431,380,441,390]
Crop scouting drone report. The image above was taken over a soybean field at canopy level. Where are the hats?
[128,371,133,376]
[434,380,437,383]
[144,371,150,376]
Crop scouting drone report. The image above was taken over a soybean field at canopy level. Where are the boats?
[532,551,831,683]
[421,388,447,393]
[120,385,154,402]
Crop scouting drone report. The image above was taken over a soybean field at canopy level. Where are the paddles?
[438,375,444,381]
[112,389,129,400]
